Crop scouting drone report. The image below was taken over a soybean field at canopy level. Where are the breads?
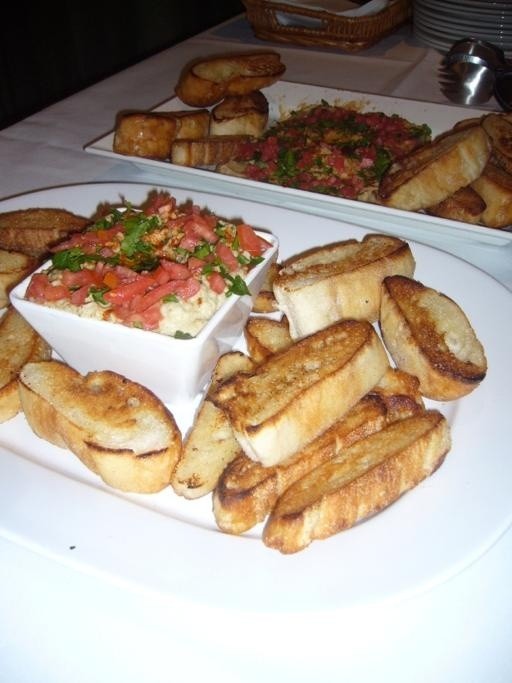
[168,233,488,557]
[1,207,183,495]
[112,52,287,167]
[377,112,512,228]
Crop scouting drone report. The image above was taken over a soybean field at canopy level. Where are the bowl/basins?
[11,207,284,410]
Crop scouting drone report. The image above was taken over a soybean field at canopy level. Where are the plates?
[412,0,512,64]
[0,180,511,598]
[82,74,510,245]
